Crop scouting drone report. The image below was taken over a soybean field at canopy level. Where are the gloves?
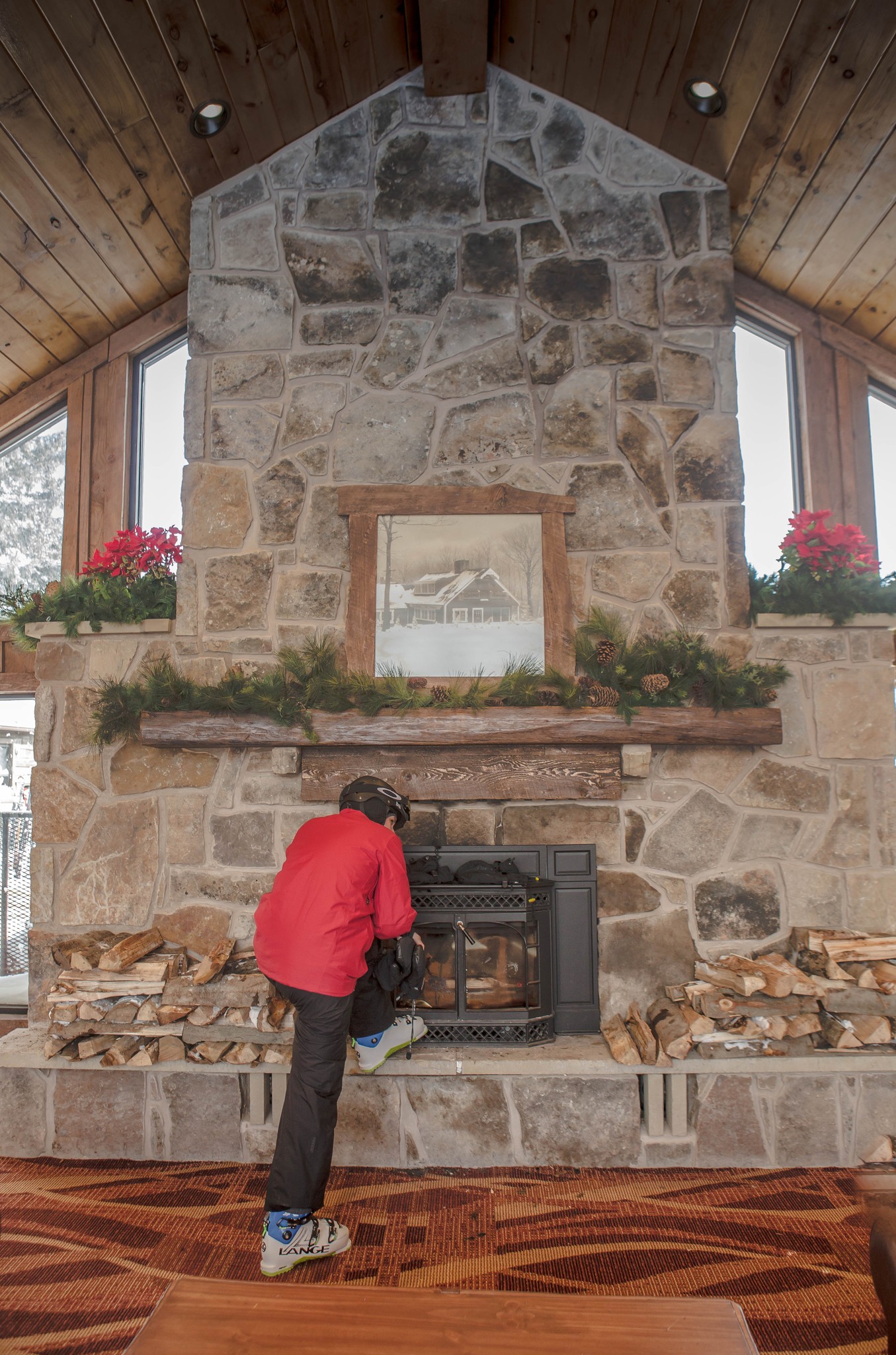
[374,936,426,1002]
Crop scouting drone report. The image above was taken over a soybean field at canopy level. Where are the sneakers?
[351,1014,428,1073]
[260,1212,351,1277]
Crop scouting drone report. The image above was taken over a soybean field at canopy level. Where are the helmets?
[339,776,407,830]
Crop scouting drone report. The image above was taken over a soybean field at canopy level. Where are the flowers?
[11,523,183,654]
[744,507,896,628]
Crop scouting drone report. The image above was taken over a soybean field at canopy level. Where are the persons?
[253,774,427,1279]
